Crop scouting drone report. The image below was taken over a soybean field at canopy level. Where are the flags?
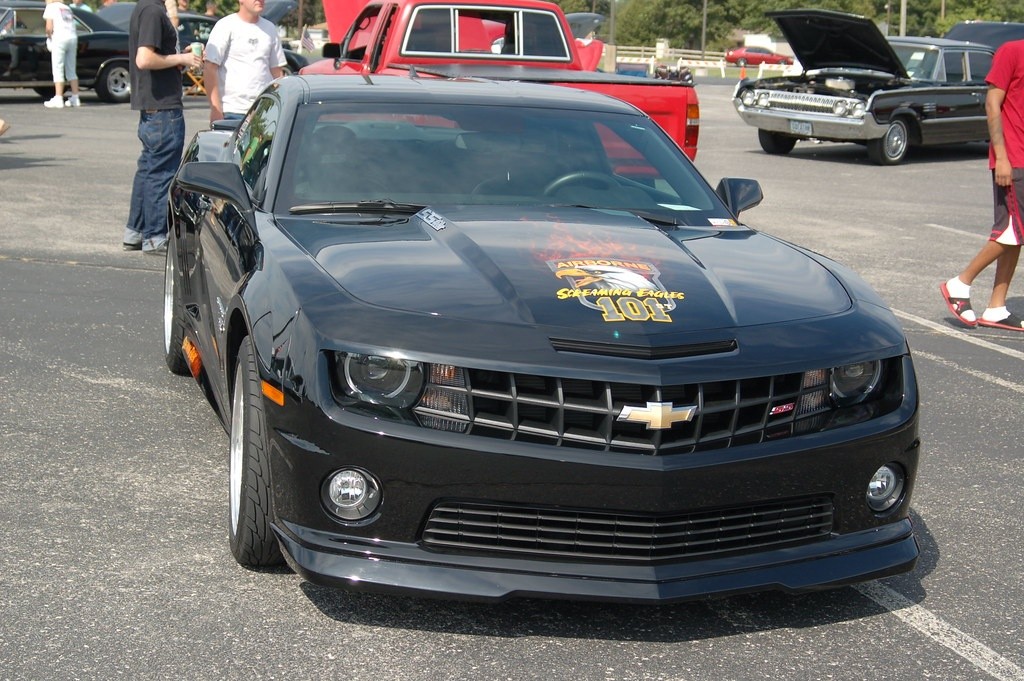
[301,27,315,52]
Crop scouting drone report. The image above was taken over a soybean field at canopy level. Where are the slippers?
[145,242,168,255]
[122,241,142,251]
[941,283,977,326]
[977,313,1024,332]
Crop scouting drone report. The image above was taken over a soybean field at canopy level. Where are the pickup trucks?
[299,0,699,162]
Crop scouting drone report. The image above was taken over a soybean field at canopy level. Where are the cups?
[190,42,203,70]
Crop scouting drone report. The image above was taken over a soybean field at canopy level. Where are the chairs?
[293,126,393,196]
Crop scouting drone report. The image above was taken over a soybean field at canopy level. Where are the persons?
[5,17,27,29]
[122,0,203,253]
[940,39,1024,332]
[42,0,81,108]
[165,0,216,39]
[97,0,118,13]
[202,0,288,129]
[69,0,92,13]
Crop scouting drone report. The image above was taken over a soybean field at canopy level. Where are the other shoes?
[64,95,81,107]
[44,98,63,108]
[1,118,10,137]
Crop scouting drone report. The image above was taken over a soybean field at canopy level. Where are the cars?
[177,0,308,87]
[0,0,130,104]
[725,46,794,67]
[164,75,921,606]
[731,9,1024,154]
